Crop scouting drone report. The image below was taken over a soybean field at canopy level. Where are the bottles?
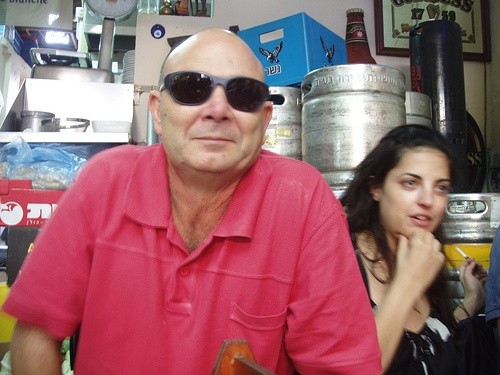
[343,8,376,66]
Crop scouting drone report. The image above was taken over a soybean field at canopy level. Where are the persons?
[0,28,383,375]
[337,124,500,375]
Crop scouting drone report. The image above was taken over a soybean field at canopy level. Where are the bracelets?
[460,301,470,317]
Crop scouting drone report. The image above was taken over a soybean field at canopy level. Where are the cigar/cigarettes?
[456,247,472,264]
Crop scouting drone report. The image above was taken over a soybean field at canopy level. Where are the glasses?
[159,71,270,112]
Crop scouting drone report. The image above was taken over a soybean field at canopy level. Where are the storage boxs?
[0,177,65,343]
[237,12,348,87]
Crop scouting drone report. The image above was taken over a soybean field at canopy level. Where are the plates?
[121,48,134,84]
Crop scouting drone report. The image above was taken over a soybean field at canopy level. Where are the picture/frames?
[374,0,492,63]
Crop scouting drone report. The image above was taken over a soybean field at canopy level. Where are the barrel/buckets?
[242,64,500,316]
